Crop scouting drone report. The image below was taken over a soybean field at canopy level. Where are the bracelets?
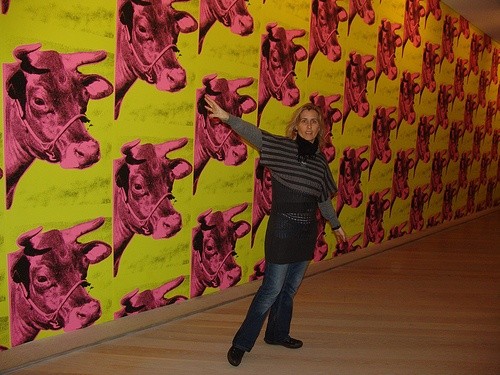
[332,225,341,230]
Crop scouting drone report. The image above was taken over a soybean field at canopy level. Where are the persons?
[204,94,349,366]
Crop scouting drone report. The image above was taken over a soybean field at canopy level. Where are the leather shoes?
[264,336,303,349]
[227,346,245,367]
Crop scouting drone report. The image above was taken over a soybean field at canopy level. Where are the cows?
[2,43,114,210]
[249,159,276,283]
[113,275,189,320]
[308,1,499,263]
[113,137,193,279]
[114,0,198,121]
[258,22,308,127]
[190,202,251,300]
[192,72,258,196]
[7,216,112,348]
[198,0,255,53]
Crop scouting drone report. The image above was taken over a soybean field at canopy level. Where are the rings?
[211,105,213,107]
